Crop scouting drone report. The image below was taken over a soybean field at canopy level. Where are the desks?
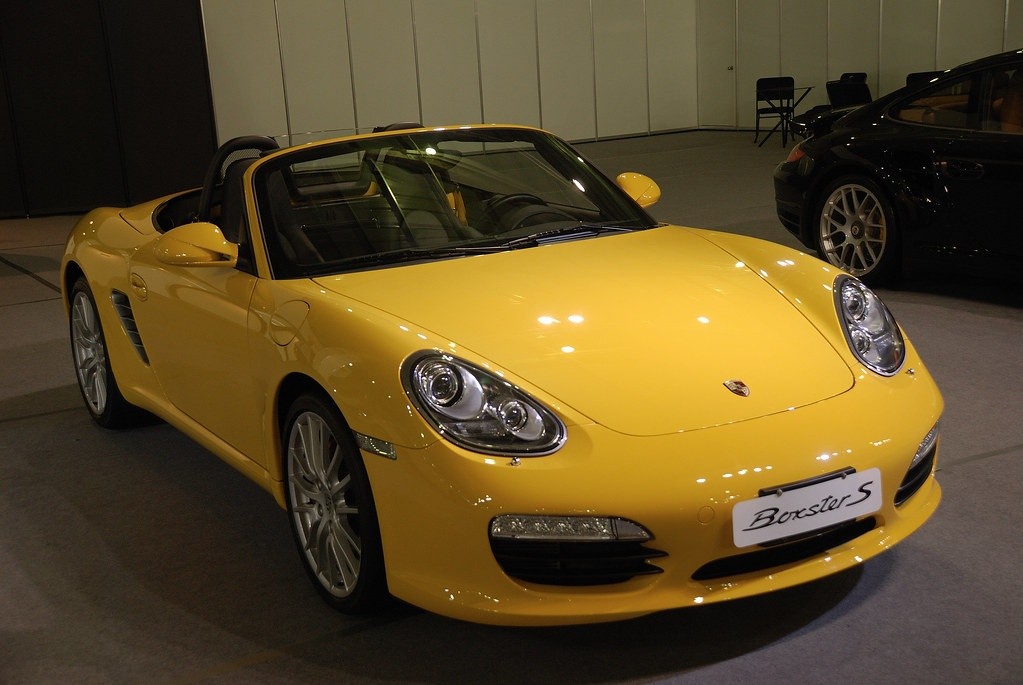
[759,86,815,148]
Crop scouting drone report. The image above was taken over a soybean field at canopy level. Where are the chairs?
[198,124,465,263]
[753,78,794,148]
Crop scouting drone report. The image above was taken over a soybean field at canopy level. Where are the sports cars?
[58,125,948,627]
[770,45,1023,286]
